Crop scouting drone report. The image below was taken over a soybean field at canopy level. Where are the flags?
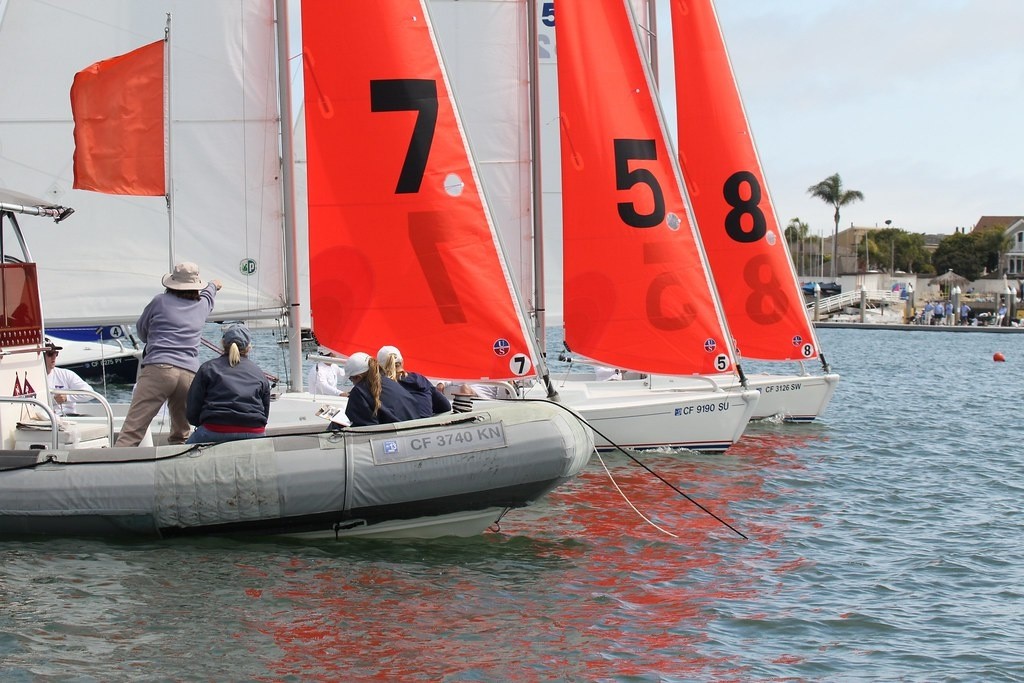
[70,39,168,197]
[891,282,900,292]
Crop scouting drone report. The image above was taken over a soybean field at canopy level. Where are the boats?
[277,325,318,352]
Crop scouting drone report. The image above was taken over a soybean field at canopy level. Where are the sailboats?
[267,0,760,455]
[43,326,142,385]
[0,1,595,542]
[542,0,838,423]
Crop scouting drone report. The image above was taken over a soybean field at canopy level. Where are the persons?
[324,352,419,430]
[376,345,451,419]
[924,300,934,325]
[995,303,1007,327]
[113,261,223,448]
[186,322,271,443]
[933,301,944,326]
[946,299,953,327]
[901,287,906,300]
[308,345,349,396]
[960,302,971,326]
[44,338,95,416]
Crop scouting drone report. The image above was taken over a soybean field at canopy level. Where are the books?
[315,403,353,426]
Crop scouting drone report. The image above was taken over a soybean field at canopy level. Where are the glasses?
[47,351,59,358]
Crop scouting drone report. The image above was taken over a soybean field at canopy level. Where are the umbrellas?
[928,270,969,301]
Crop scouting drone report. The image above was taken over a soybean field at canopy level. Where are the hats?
[162,262,208,290]
[224,324,252,350]
[343,352,371,383]
[44,334,63,351]
[377,345,402,364]
[318,346,332,356]
